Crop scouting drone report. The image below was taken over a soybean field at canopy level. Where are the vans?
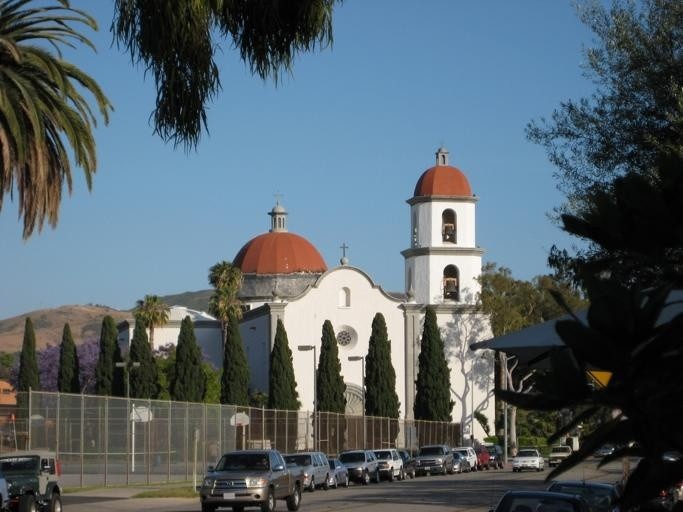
[282,451,331,491]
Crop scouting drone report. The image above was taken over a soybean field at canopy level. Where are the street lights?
[298,345,319,450]
[348,355,367,449]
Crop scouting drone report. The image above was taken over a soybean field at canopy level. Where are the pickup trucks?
[200,450,301,512]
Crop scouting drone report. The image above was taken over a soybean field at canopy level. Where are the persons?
[512,446,517,458]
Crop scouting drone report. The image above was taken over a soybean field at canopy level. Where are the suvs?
[341,443,504,484]
[0,450,63,512]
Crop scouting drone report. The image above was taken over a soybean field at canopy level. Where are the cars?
[327,458,350,489]
[488,479,622,512]
[600,446,612,457]
[513,449,545,472]
[549,445,573,467]
